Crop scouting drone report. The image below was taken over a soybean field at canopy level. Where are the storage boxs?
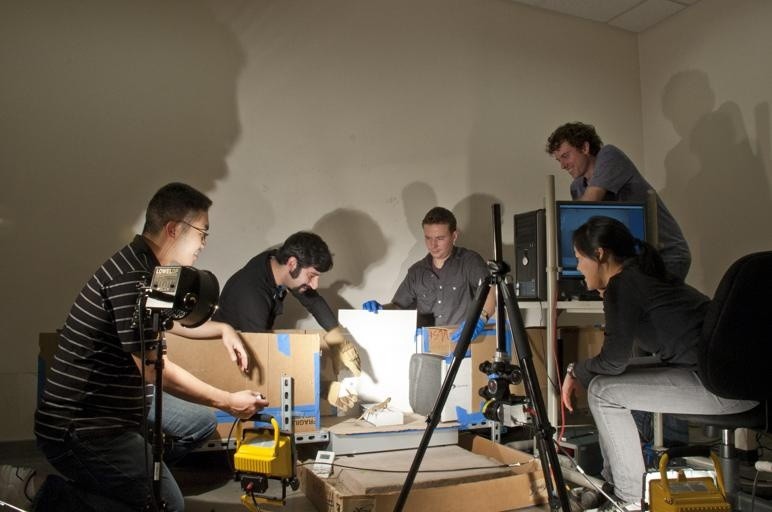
[38,330,319,433]
[296,431,571,512]
[423,323,605,422]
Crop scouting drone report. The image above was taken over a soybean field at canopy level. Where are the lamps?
[640,445,732,511]
[141,266,219,512]
[229,414,297,508]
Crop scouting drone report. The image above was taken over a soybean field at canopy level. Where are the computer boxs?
[512,207,544,301]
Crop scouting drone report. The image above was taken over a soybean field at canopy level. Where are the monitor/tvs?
[556,199,649,301]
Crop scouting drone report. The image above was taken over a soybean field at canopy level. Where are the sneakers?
[584,496,646,512]
[588,481,614,508]
[0,465,37,512]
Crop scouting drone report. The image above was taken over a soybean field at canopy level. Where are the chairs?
[672,249,772,511]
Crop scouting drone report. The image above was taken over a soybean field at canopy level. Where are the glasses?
[174,219,211,244]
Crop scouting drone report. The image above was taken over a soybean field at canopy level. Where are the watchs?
[566,361,577,379]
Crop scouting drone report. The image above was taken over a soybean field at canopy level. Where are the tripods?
[391,202,571,512]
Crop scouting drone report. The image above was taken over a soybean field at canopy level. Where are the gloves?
[450,317,486,343]
[362,300,383,313]
[324,327,363,377]
[320,378,359,412]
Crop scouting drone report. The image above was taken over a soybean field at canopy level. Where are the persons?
[545,121,692,283]
[361,206,498,345]
[0,181,272,511]
[210,230,361,411]
[560,215,763,512]
